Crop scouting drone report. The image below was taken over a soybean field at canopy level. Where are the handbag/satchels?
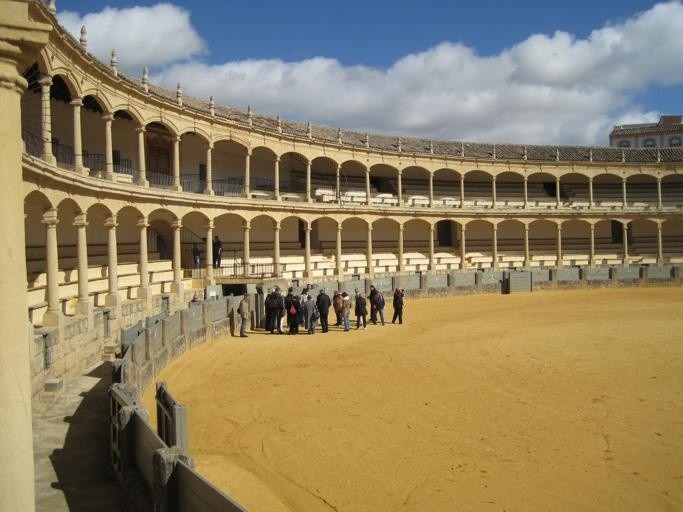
[289,304,297,314]
[312,311,318,320]
[237,308,240,314]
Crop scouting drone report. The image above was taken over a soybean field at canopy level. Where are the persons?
[240,294,250,337]
[212,236,222,268]
[193,243,200,268]
[191,295,201,302]
[392,288,404,324]
[265,284,385,334]
